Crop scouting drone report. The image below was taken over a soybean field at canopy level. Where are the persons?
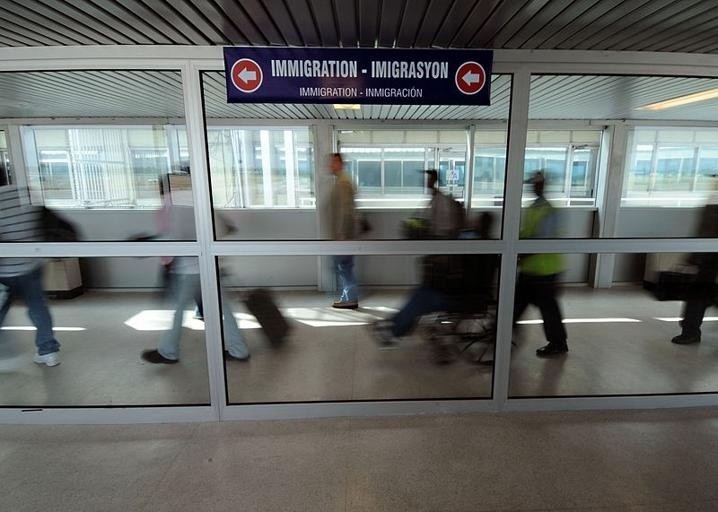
[398,169,467,267]
[366,211,501,347]
[670,171,718,346]
[1,162,64,366]
[317,151,372,309]
[493,168,569,356]
[125,158,251,363]
[151,169,203,320]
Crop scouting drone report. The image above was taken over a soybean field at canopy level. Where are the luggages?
[221,267,288,348]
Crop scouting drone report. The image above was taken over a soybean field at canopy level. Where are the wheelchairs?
[420,255,524,370]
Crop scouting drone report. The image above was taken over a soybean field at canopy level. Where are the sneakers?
[224,350,249,362]
[536,339,568,356]
[142,350,178,364]
[33,352,61,367]
[671,327,701,343]
[332,298,358,309]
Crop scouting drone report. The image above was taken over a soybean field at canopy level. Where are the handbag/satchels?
[654,272,696,301]
[43,206,77,241]
